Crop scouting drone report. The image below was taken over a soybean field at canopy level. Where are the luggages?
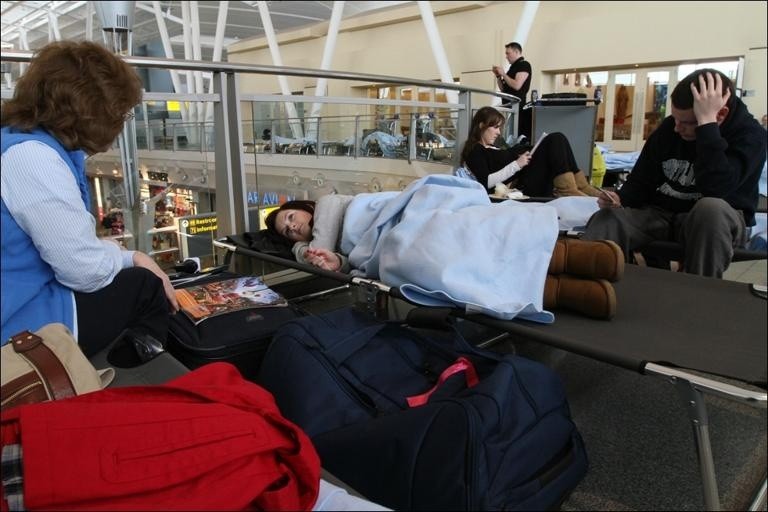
[170,271,303,369]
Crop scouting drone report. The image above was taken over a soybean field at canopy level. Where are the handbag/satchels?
[255,304,588,512]
[1,323,115,411]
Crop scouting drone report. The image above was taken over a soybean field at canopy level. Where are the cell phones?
[598,186,615,203]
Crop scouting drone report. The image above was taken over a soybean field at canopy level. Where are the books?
[529,131,548,156]
[173,273,290,326]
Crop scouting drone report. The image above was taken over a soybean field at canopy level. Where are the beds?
[601,151,767,211]
[487,192,766,276]
[211,229,768,511]
[131,131,463,171]
[0,332,393,512]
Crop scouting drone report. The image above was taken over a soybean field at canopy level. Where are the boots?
[538,239,625,320]
[554,170,603,200]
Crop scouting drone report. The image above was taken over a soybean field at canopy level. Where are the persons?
[263,193,627,321]
[490,42,532,111]
[760,115,768,132]
[1,37,180,354]
[577,69,768,280]
[462,106,608,202]
